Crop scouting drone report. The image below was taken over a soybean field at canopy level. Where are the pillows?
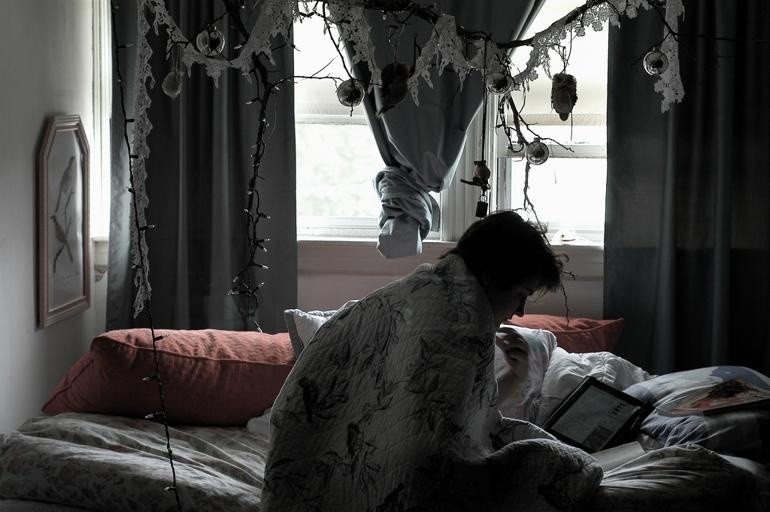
[624,366,770,454]
[502,313,625,354]
[283,307,337,360]
[40,327,298,430]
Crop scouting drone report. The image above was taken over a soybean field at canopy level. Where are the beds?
[0,349,770,512]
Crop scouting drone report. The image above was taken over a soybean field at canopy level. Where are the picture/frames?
[34,112,91,331]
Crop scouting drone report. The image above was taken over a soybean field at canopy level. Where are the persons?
[436,208,568,408]
